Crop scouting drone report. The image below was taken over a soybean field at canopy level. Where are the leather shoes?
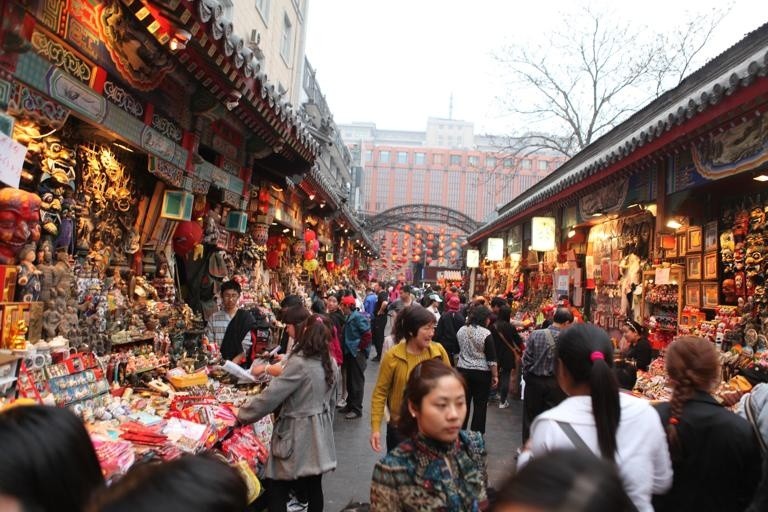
[345,411,358,419]
[373,356,380,362]
[338,404,351,414]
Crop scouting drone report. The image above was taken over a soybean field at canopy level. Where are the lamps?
[466,248,480,268]
[531,216,556,252]
[487,237,504,260]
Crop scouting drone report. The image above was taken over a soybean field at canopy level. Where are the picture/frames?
[662,222,720,309]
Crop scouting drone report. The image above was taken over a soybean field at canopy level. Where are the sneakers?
[336,398,347,407]
[487,393,510,409]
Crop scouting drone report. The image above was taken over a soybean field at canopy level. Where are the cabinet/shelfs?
[638,264,685,334]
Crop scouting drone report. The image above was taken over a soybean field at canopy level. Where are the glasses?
[223,294,238,301]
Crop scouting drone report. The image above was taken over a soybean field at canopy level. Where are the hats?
[429,294,443,303]
[340,296,356,306]
[446,297,460,309]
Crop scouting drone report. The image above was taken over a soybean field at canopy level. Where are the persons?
[0,187,77,338]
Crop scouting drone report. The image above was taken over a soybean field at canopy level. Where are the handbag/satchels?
[512,347,522,375]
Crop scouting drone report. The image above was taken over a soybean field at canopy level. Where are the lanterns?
[372,223,468,270]
[303,229,320,281]
